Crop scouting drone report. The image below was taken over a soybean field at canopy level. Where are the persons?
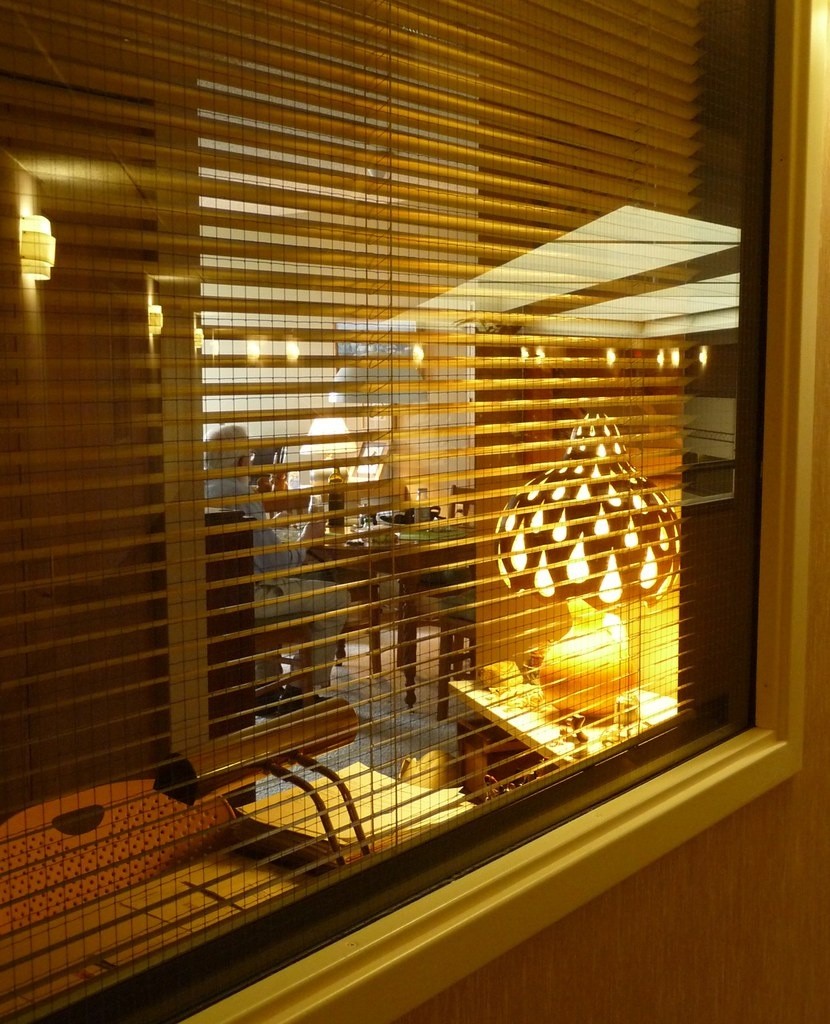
[207,425,350,712]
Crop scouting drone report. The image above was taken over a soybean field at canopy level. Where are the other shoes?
[254,682,333,716]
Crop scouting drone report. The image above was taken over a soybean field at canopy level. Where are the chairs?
[402,484,475,720]
[256,609,315,713]
[259,496,377,672]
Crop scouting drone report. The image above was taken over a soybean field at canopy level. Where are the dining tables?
[264,511,480,708]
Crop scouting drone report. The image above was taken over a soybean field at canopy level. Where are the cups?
[308,495,326,524]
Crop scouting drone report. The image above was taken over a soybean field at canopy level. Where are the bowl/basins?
[523,660,542,685]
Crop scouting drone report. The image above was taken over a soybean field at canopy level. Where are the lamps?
[493,414,682,719]
[19,214,54,282]
[243,333,711,373]
[195,326,203,346]
[300,417,356,484]
[146,304,163,333]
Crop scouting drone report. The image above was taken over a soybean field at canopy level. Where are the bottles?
[415,488,430,522]
[328,467,344,527]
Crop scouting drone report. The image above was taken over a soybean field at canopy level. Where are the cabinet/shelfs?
[204,504,257,739]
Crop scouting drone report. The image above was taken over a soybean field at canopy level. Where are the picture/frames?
[333,318,423,380]
[346,438,390,482]
